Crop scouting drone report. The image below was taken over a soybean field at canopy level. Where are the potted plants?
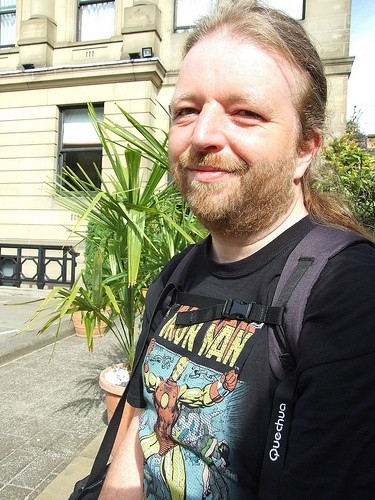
[30,101,209,428]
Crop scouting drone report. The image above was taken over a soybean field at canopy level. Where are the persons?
[97,0,375,500]
